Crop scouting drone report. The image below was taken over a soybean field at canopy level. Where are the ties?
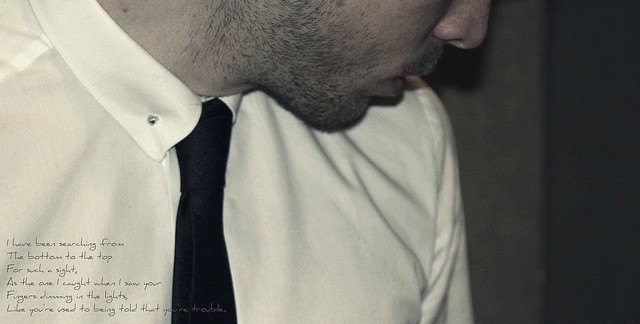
[171,97,237,324]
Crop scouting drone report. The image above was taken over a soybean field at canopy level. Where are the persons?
[0,0,493,324]
